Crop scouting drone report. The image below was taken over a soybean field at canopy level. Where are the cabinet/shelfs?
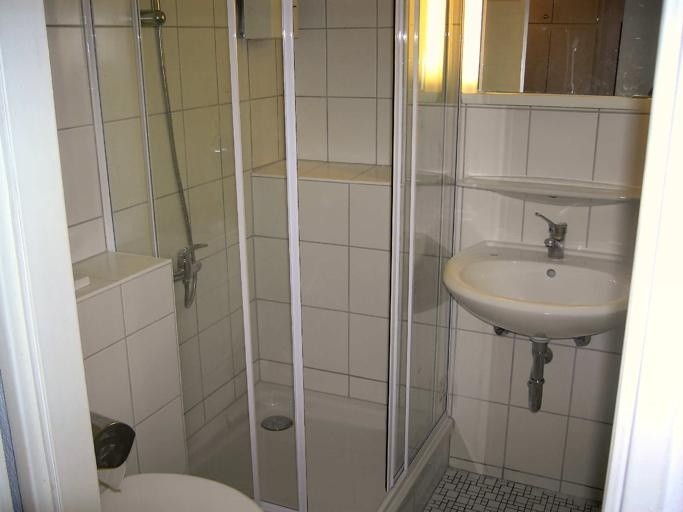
[526,0,606,94]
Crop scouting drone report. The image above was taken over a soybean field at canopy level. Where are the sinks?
[444,241,632,342]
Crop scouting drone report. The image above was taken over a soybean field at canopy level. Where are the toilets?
[99,473,264,512]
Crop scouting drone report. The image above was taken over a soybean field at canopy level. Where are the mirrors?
[479,0,664,97]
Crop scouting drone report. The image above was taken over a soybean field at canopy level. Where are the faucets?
[534,213,567,260]
